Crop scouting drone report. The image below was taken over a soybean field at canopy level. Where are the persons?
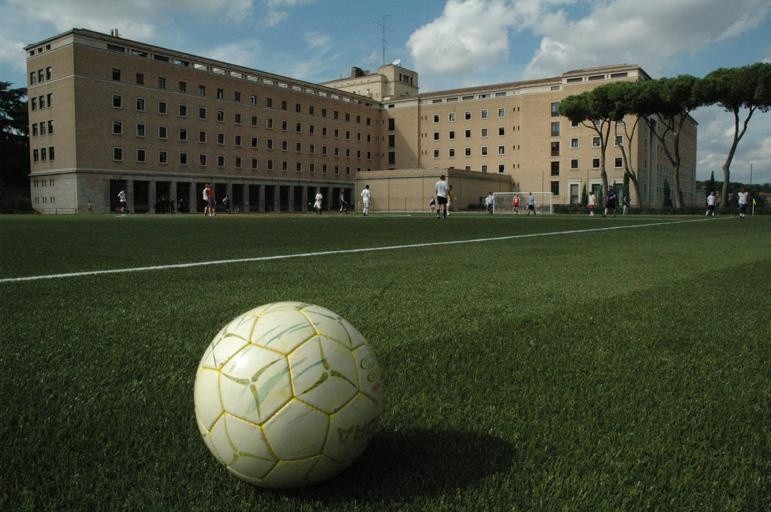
[206,184,216,216]
[527,192,537,216]
[312,190,324,215]
[623,192,632,215]
[222,194,231,214]
[430,197,436,214]
[202,184,209,216]
[479,192,484,211]
[124,188,128,194]
[587,191,597,216]
[737,186,751,220]
[603,185,617,218]
[512,192,521,216]
[447,185,458,216]
[154,195,189,214]
[88,199,93,214]
[118,187,130,214]
[486,192,493,216]
[435,174,449,219]
[361,185,372,216]
[704,190,716,217]
[338,191,350,216]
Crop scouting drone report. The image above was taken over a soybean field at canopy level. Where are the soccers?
[193,301,382,488]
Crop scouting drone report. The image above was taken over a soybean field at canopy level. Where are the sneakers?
[203,212,217,217]
[488,212,493,216]
[740,215,745,219]
[436,212,450,220]
